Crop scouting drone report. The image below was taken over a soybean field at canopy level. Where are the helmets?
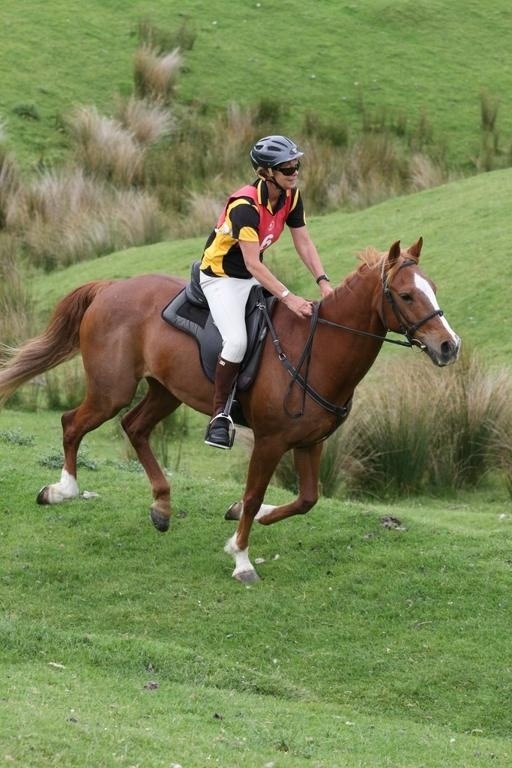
[250,134,305,171]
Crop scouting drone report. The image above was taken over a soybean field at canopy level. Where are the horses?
[0,234,463,586]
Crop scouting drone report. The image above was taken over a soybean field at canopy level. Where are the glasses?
[277,164,301,176]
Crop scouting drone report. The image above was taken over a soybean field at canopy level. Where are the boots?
[208,354,244,445]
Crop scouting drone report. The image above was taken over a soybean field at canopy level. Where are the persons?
[199,134,336,451]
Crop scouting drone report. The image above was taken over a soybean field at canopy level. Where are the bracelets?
[277,288,291,302]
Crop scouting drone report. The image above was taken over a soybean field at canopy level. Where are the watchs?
[316,273,330,285]
